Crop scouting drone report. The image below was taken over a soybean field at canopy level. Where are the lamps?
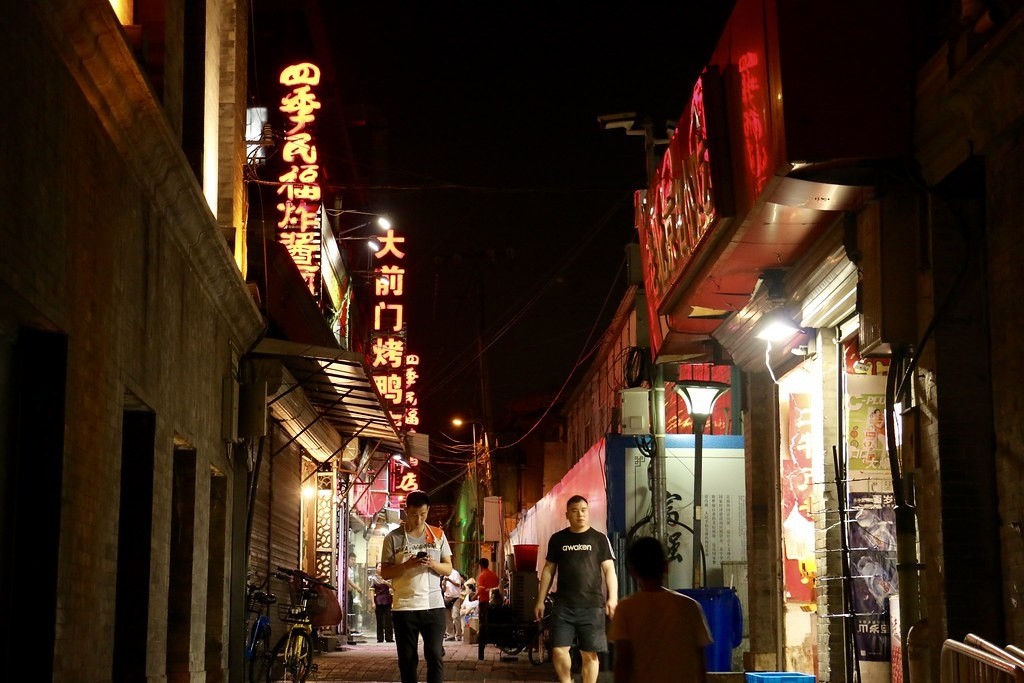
[326,209,394,229]
[336,235,381,252]
[352,271,389,285]
[754,305,802,342]
[791,346,808,356]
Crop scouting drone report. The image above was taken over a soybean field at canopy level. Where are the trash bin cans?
[674,588,743,672]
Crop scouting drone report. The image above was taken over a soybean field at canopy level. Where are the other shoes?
[377,639,383,643]
[386,639,395,642]
[457,635,462,641]
[445,636,455,641]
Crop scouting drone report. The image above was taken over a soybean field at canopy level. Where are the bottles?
[858,557,899,610]
[472,614,479,617]
[465,615,470,624]
[855,509,897,551]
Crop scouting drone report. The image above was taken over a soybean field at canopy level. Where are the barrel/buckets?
[513,545,539,571]
[506,555,514,572]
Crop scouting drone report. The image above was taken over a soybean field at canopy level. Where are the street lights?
[666,378,736,591]
[453,417,483,577]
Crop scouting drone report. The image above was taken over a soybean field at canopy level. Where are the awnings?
[248,338,404,512]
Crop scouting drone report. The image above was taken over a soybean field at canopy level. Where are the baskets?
[248,595,266,613]
[278,603,315,625]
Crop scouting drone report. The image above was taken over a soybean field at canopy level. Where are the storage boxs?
[745,672,817,683]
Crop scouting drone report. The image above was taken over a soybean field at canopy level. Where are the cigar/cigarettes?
[534,619,540,622]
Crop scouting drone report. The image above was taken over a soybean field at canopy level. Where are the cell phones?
[416,552,427,562]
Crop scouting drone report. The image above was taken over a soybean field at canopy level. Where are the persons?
[607,536,715,683]
[347,553,363,637]
[442,558,499,641]
[534,495,618,683]
[370,562,395,643]
[381,491,453,683]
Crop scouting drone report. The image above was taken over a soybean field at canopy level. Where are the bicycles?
[241,565,338,683]
[495,591,556,666]
